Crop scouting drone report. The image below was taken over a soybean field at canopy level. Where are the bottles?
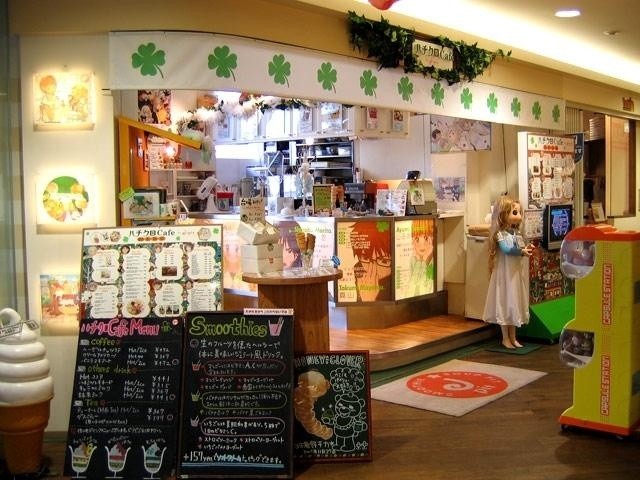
[298,147,313,156]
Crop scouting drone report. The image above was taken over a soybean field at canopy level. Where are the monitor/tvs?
[543,204,572,250]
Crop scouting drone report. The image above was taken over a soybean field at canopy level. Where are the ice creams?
[72,441,94,472]
[109,442,125,470]
[145,442,161,472]
[0,307,54,474]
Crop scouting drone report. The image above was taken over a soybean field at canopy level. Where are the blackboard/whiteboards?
[63,315,183,480]
[296,350,374,464]
[177,311,295,480]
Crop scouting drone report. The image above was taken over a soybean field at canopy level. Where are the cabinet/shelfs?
[292,140,355,184]
[150,168,218,213]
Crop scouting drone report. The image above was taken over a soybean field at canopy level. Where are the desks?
[242,268,343,352]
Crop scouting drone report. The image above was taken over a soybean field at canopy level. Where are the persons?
[278,221,307,268]
[350,222,392,302]
[39,75,57,123]
[88,282,97,291]
[482,198,534,349]
[184,243,194,252]
[410,220,436,298]
[154,244,162,253]
[184,280,193,290]
[121,245,130,254]
[431,118,491,151]
[152,280,162,290]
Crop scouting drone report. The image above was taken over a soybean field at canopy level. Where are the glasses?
[358,253,392,267]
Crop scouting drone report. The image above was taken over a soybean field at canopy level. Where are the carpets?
[370,358,549,417]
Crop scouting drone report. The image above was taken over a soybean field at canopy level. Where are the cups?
[168,201,178,217]
[160,203,167,218]
